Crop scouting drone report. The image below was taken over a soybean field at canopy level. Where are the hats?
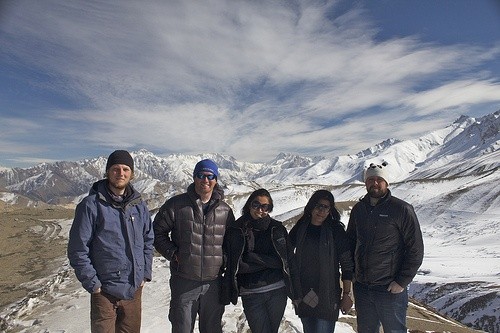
[106,150,134,173]
[194,159,218,177]
[364,162,390,186]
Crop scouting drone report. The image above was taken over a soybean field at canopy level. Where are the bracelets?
[343,292,349,295]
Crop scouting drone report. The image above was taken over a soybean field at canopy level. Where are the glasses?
[312,202,331,213]
[193,173,216,180]
[251,201,272,211]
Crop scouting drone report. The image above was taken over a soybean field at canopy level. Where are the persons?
[152,159,235,333]
[67,150,154,333]
[289,190,353,333]
[347,163,424,333]
[235,189,299,333]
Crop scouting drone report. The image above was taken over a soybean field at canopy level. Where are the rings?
[401,289,403,292]
